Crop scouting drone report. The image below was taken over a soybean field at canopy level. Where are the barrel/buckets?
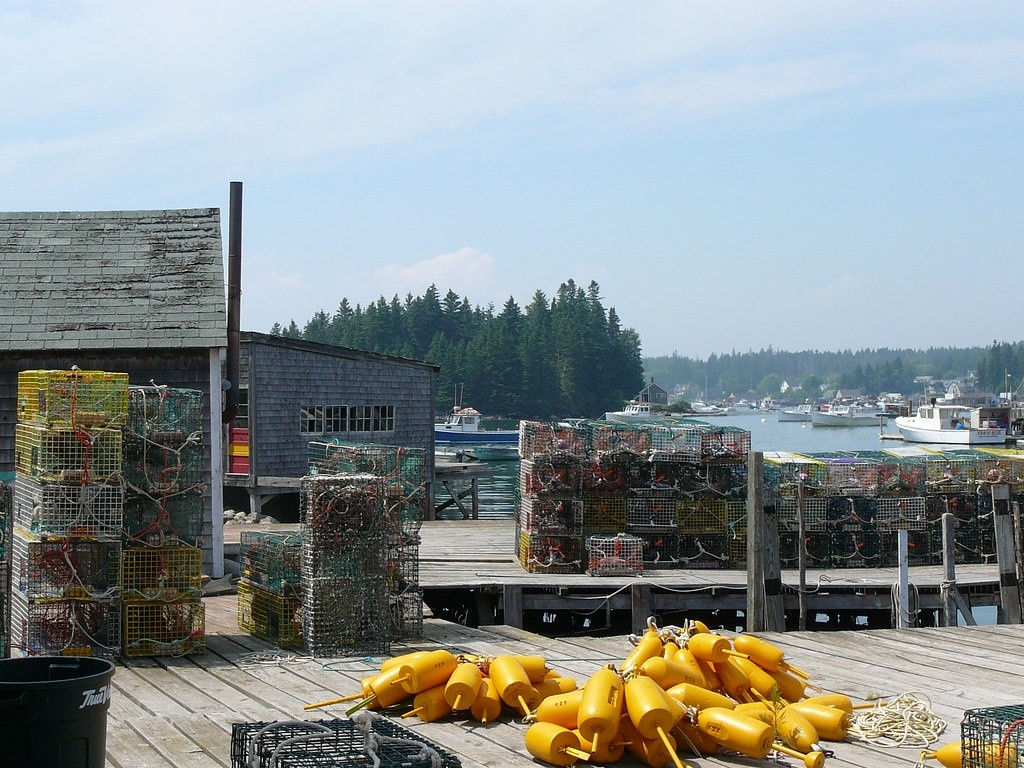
[0,656,116,768]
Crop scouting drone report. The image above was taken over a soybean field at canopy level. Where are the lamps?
[221,378,232,392]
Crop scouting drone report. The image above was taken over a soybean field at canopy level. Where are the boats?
[809,399,889,427]
[459,443,520,461]
[893,378,1007,446]
[603,385,684,424]
[687,365,906,423]
[433,446,489,474]
[433,381,520,446]
